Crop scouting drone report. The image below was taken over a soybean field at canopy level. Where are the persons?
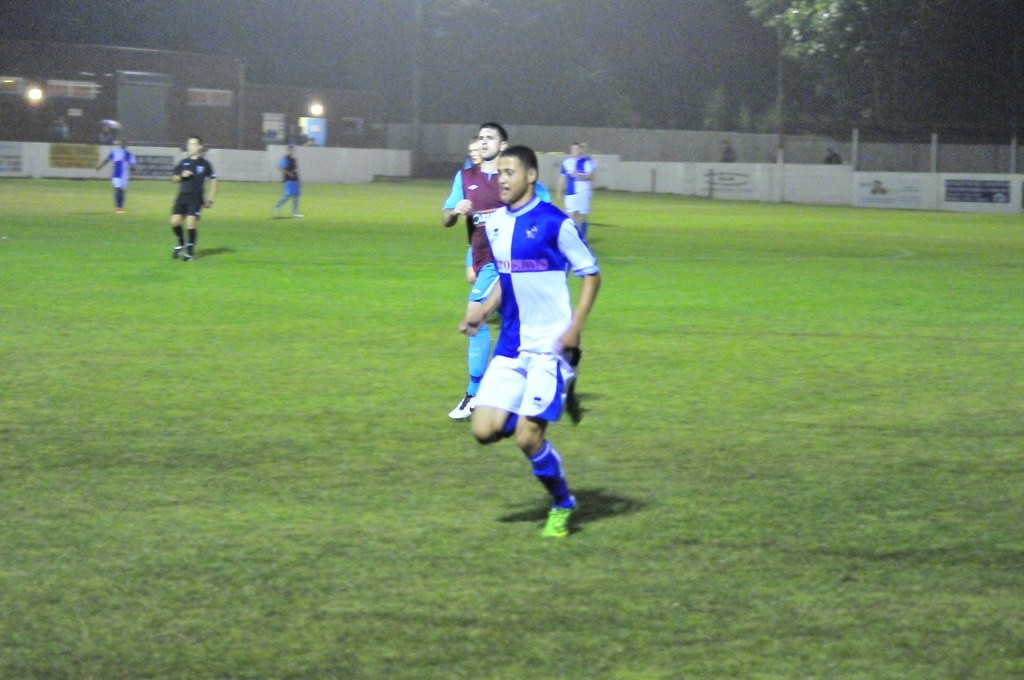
[93,126,115,145]
[169,137,218,259]
[720,140,737,163]
[296,126,319,147]
[52,115,73,142]
[464,136,485,284]
[459,145,603,539]
[556,141,599,238]
[273,145,307,218]
[442,123,554,420]
[825,146,843,164]
[95,138,138,213]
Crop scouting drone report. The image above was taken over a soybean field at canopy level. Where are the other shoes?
[117,207,124,212]
[273,206,278,217]
[293,215,304,217]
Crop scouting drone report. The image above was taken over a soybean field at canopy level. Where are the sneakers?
[184,255,196,261]
[174,246,183,258]
[544,498,580,538]
[448,394,477,419]
[564,386,584,426]
